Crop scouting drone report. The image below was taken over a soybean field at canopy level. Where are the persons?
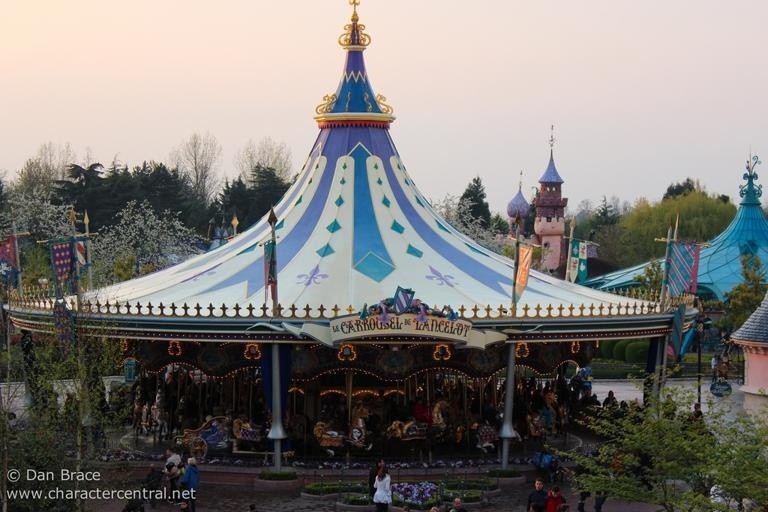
[122,355,728,512]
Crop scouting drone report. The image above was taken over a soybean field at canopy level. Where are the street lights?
[694,317,713,418]
